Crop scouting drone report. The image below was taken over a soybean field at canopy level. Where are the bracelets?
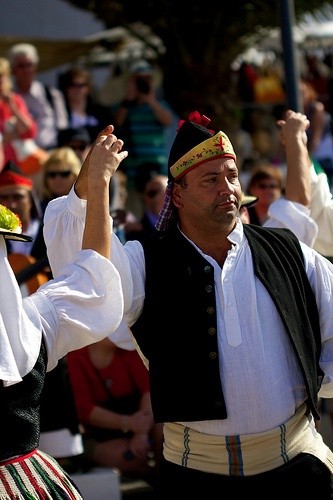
[122,415,129,434]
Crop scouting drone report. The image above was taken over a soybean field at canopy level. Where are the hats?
[0,160,43,220]
[59,127,96,144]
[154,110,237,240]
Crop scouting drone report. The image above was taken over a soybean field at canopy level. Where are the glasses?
[44,170,70,178]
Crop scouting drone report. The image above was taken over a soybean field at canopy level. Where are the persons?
[42,110,333,500]
[0,133,129,500]
[0,44,333,481]
